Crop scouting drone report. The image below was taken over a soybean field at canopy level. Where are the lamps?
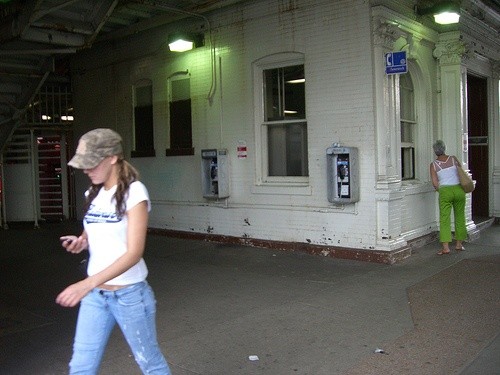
[418,0,460,25]
[167,32,205,53]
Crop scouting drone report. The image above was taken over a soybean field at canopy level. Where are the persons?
[56,128,172,375]
[430,140,468,255]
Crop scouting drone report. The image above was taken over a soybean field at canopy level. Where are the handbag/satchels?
[452,155,475,193]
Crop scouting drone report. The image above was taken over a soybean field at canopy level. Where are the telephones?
[210,166,216,179]
[337,164,349,180]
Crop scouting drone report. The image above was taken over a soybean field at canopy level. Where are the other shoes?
[436,249,450,255]
[456,246,465,250]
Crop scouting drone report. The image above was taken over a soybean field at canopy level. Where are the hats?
[67,128,123,170]
